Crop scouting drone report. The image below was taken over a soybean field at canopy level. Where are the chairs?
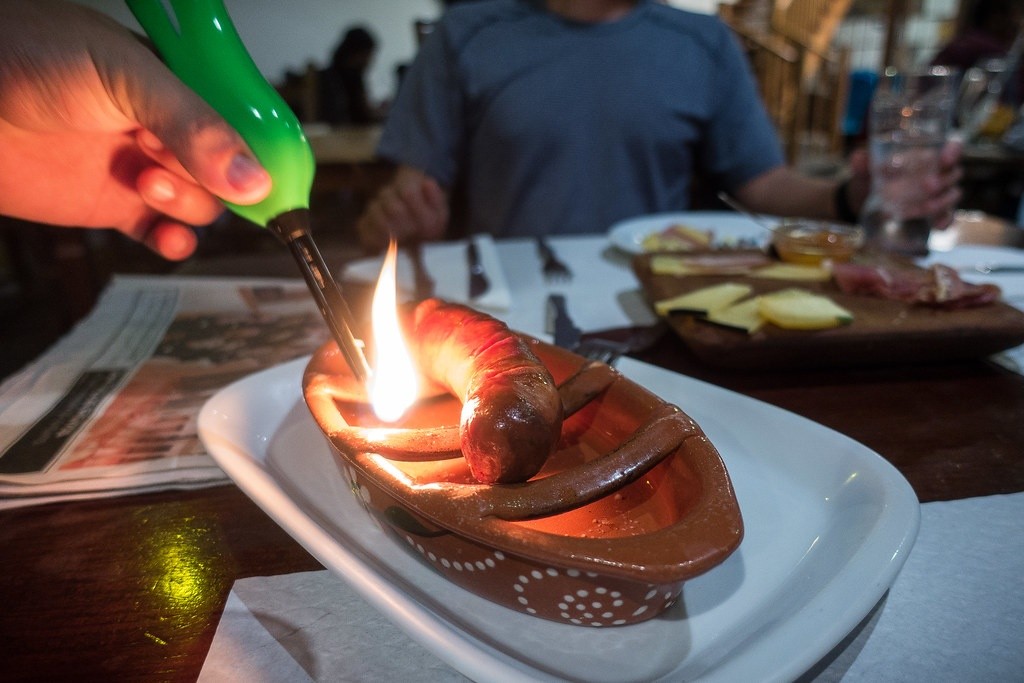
[718,0,854,170]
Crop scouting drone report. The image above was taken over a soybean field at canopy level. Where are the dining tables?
[3,239,1022,683]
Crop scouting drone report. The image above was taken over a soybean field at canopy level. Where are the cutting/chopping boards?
[630,250,1024,364]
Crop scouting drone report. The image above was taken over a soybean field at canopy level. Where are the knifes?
[550,294,584,350]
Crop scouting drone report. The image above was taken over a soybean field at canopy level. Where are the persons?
[156,284,322,363]
[310,26,393,131]
[0,1,273,262]
[353,0,961,257]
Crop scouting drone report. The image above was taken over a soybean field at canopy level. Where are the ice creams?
[390,297,563,484]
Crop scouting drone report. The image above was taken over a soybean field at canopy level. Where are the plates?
[607,211,781,256]
[302,333,745,584]
[197,336,922,683]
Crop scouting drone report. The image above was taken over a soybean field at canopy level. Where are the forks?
[535,233,573,286]
[574,329,659,366]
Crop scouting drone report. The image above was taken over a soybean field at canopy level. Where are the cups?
[773,221,863,268]
[865,73,949,254]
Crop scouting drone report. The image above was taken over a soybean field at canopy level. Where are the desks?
[304,126,383,238]
